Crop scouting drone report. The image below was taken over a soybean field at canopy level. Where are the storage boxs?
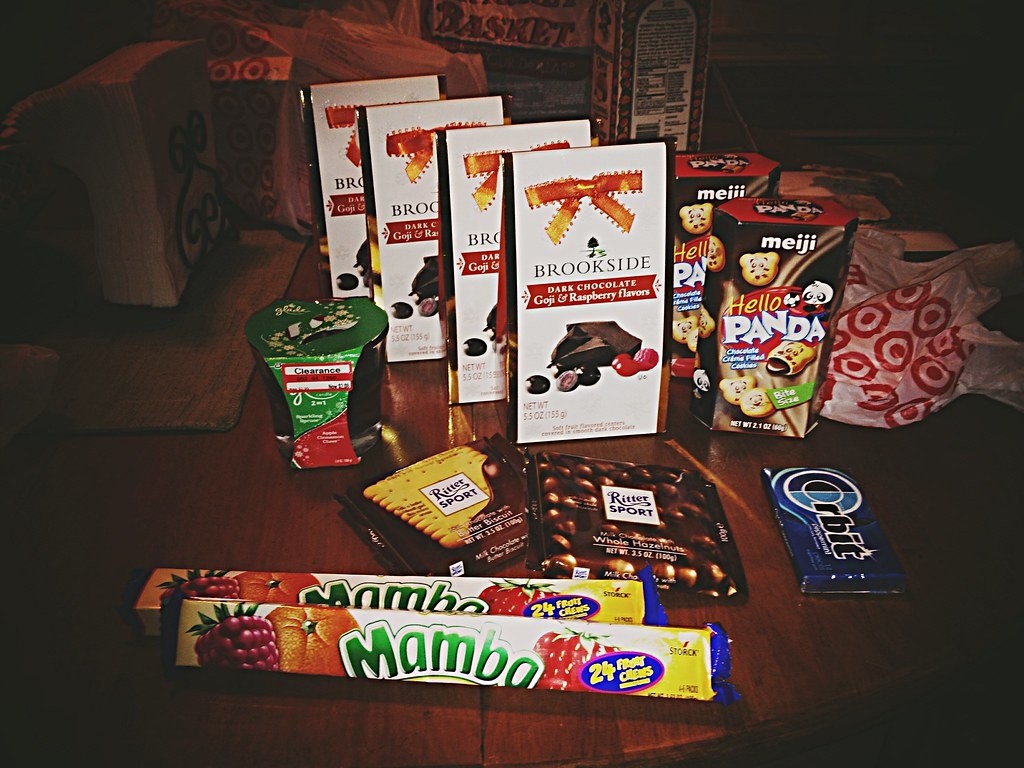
[690,194,862,438]
[760,466,907,597]
[669,149,781,377]
